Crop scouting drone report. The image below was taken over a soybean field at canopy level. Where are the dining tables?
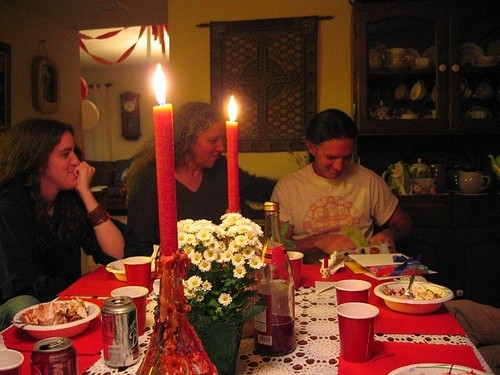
[0,263,492,375]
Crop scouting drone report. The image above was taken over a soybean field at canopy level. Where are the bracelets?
[84,202,111,228]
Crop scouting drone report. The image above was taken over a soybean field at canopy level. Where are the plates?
[373,280,456,315]
[386,360,490,375]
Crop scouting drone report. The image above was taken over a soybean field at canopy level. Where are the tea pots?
[456,170,490,195]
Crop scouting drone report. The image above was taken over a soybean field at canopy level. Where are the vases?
[190,315,243,375]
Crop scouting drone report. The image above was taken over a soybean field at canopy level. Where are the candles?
[153,62,178,256]
[225,95,240,214]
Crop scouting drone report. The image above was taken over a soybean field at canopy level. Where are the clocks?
[120,91,142,140]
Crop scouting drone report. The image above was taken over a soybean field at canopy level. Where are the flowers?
[174,213,266,323]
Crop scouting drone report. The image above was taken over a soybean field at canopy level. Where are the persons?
[269,108,415,253]
[122,101,278,259]
[0,118,141,332]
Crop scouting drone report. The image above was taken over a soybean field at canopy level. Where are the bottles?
[409,158,445,193]
[255,200,297,355]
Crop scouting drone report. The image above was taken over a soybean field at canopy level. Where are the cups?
[368,41,439,119]
[0,348,25,375]
[335,300,379,364]
[335,279,372,309]
[284,250,303,290]
[124,255,151,293]
[110,285,149,337]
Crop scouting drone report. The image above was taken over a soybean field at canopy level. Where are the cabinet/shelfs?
[350,0,500,309]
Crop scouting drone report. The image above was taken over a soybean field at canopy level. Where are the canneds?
[30,336,79,375]
[101,296,140,367]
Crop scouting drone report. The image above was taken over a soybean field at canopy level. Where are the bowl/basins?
[13,299,101,342]
[106,257,159,283]
